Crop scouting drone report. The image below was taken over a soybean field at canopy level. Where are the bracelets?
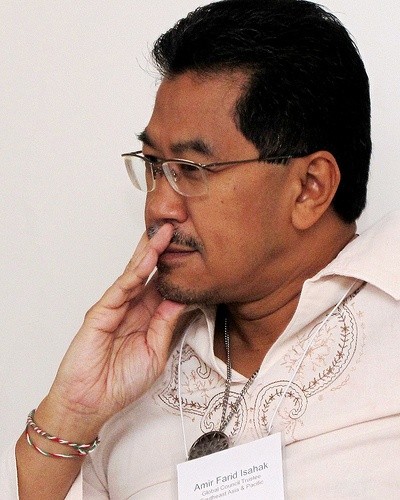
[25,410,103,458]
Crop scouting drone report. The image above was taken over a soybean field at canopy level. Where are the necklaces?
[189,312,261,460]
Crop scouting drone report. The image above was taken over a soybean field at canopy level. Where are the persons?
[16,0,398,500]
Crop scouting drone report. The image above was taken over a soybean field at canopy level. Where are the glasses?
[121,150,292,196]
